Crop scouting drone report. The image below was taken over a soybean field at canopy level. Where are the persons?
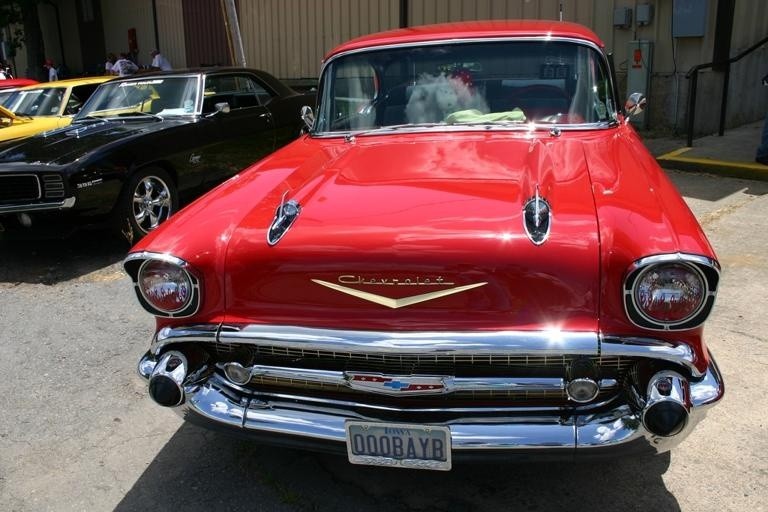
[103,51,141,76]
[148,48,171,71]
[44,58,58,81]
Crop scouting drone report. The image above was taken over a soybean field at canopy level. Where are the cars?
[0,60,317,244]
[0,78,42,91]
[114,16,727,481]
[0,73,217,145]
